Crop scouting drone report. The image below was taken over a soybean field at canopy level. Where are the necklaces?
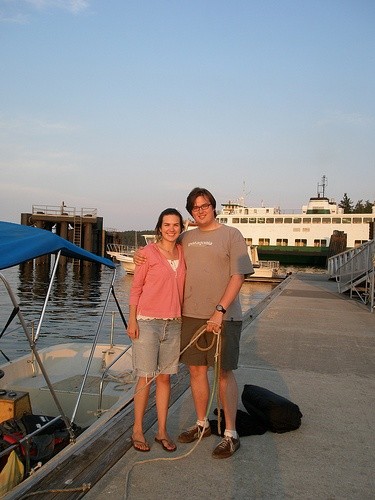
[157,245,177,265]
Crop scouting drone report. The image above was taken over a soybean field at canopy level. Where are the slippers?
[131,434,150,452]
[155,436,176,452]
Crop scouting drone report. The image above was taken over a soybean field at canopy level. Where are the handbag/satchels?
[208,408,267,438]
[241,384,303,434]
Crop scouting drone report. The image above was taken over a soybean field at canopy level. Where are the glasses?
[192,203,212,212]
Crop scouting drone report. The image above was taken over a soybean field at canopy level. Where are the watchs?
[216,305,227,314]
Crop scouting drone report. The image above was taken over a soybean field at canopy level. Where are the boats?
[106,235,286,283]
[0,221,133,496]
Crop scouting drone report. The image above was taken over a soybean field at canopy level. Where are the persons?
[133,187,255,459]
[126,208,187,452]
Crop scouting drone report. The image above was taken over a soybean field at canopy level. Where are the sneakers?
[212,435,240,459]
[178,424,212,443]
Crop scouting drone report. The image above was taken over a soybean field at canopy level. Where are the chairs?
[104,348,133,371]
